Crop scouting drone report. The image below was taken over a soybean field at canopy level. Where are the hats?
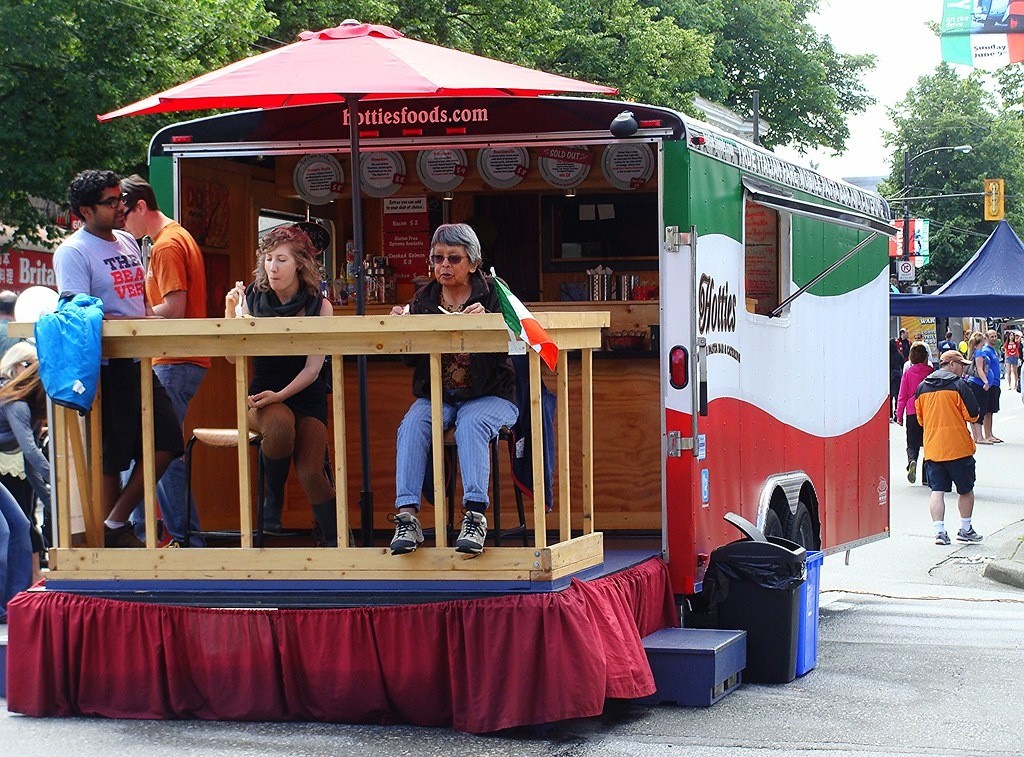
[0,289,18,304]
[939,350,973,365]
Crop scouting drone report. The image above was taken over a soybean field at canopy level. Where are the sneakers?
[103,521,146,548]
[955,524,983,544]
[389,512,424,555]
[454,511,487,553]
[935,531,951,545]
[908,460,916,483]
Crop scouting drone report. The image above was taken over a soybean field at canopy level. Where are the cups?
[587,273,612,301]
[616,274,639,301]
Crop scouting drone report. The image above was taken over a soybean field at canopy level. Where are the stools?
[181,430,339,551]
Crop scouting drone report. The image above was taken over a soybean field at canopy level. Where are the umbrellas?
[90,14,624,547]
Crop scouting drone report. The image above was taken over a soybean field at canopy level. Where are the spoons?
[234,280,243,317]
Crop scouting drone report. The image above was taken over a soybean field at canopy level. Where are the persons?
[0,359,51,635]
[386,222,528,557]
[881,321,1024,484]
[50,168,180,550]
[1,286,21,354]
[107,174,207,550]
[913,349,987,547]
[222,220,356,547]
[0,342,38,387]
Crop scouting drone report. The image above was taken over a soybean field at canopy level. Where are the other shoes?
[974,437,1004,445]
[1000,376,1005,378]
[894,411,898,422]
[890,418,893,423]
[1008,386,1012,390]
[1014,386,1018,389]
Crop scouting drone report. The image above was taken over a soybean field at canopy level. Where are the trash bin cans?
[707,512,807,685]
[796,551,826,678]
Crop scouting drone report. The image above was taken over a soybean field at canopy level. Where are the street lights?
[904,146,973,295]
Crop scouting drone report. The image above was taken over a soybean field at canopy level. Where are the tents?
[889,218,1024,319]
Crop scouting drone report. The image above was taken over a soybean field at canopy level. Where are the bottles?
[362,253,396,305]
[319,266,328,298]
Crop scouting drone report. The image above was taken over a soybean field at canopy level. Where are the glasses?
[94,192,128,209]
[430,254,470,264]
[914,337,921,339]
[122,203,137,222]
[989,335,996,338]
[20,362,33,368]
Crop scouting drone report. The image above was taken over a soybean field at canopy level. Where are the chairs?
[432,356,530,548]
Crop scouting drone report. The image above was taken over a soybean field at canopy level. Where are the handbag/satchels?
[962,349,989,379]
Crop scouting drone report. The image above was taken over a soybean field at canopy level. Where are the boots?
[312,497,337,546]
[262,449,291,532]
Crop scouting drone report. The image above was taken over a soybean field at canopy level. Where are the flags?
[494,276,560,374]
